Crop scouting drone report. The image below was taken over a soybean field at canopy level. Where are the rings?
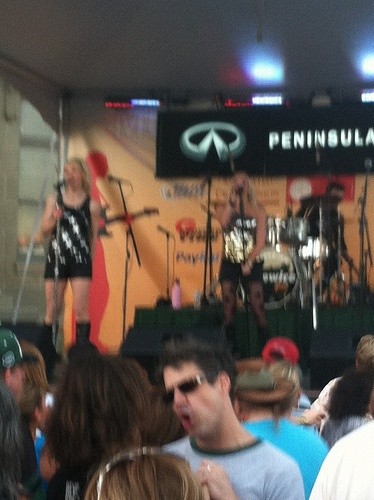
[206,464,212,473]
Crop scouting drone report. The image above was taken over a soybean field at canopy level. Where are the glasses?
[163,377,204,403]
[97,449,191,500]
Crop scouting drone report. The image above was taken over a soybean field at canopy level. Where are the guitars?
[97,204,159,234]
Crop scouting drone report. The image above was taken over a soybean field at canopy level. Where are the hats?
[262,337,300,365]
[0,328,39,372]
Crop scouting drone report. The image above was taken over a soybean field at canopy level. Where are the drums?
[267,217,285,246]
[284,216,309,245]
[298,236,329,259]
[240,247,301,310]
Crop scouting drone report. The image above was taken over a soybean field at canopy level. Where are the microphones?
[108,175,124,182]
[236,186,244,194]
[157,225,175,240]
[54,179,66,190]
[201,166,217,185]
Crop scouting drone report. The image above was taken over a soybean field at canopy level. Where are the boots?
[76,322,91,343]
[225,325,239,355]
[257,327,269,351]
[44,321,59,346]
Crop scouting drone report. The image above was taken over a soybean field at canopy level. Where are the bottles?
[172,278,182,308]
[194,288,203,311]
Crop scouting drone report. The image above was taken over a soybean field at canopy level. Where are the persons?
[41,157,99,350]
[215,172,269,354]
[297,180,348,303]
[0,328,374,500]
